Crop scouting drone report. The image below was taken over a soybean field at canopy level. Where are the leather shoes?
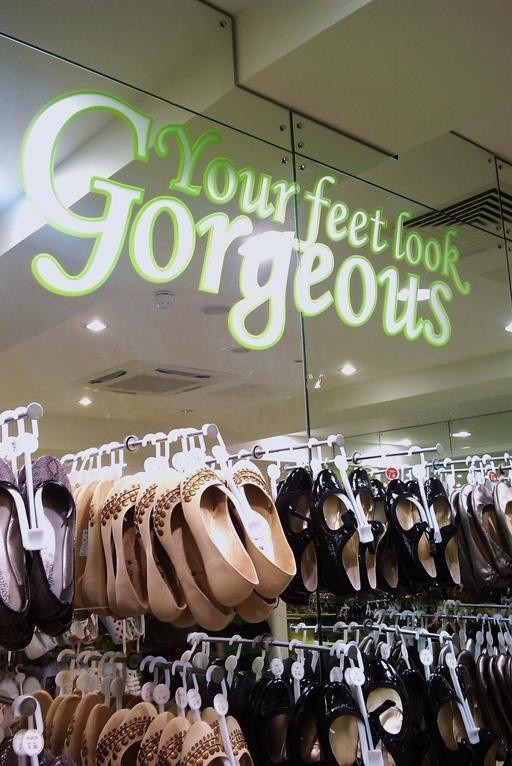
[0,456,512,765]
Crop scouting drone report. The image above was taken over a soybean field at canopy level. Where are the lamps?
[83,318,110,334]
